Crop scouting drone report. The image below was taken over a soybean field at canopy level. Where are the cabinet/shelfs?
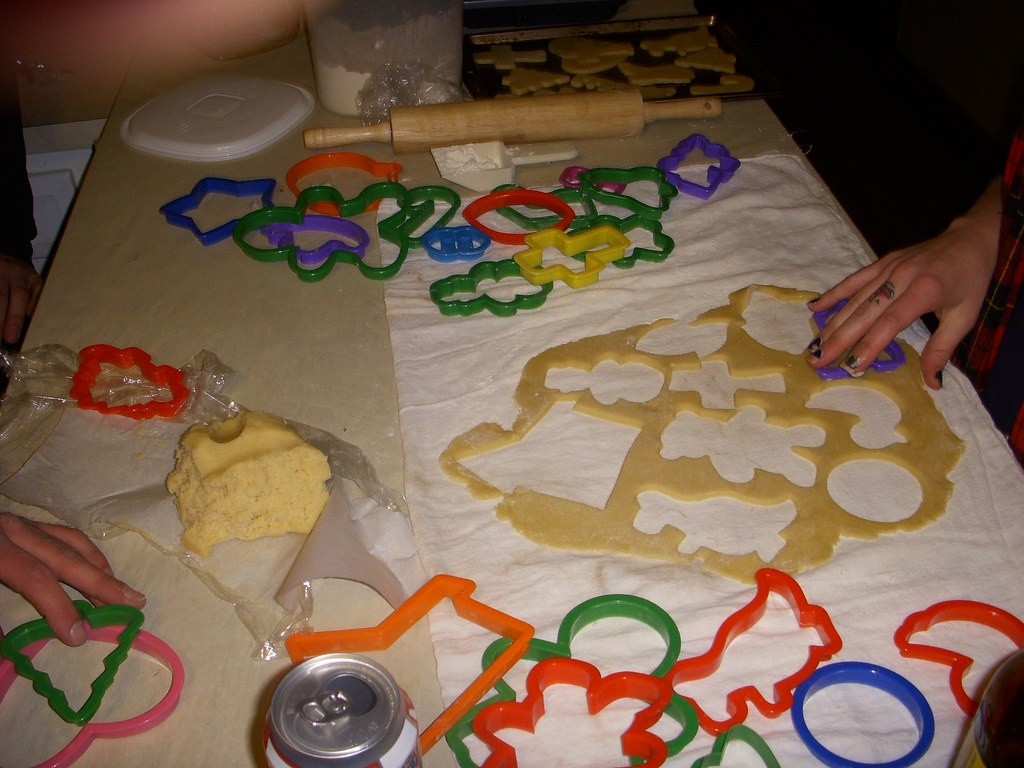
[24,169,77,278]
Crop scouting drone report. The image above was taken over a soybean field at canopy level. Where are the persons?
[0,60,148,647]
[808,117,1024,470]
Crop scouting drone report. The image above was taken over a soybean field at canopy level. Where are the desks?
[1,0,1024,767]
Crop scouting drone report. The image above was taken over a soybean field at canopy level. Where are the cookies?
[471,26,754,101]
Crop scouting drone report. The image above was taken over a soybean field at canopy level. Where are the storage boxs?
[304,0,466,118]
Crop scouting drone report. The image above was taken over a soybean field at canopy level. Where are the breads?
[165,411,333,558]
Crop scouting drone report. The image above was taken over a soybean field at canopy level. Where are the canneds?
[261,652,425,768]
